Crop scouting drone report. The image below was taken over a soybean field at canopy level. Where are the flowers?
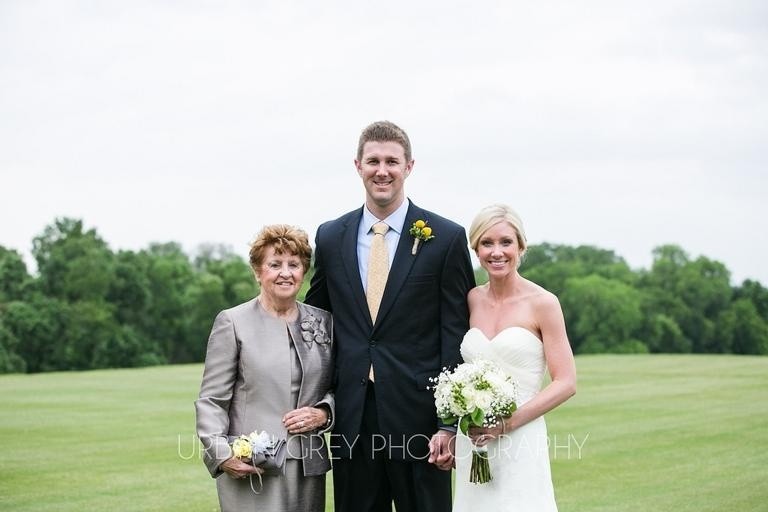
[409,218,435,256]
[427,356,518,483]
[231,429,272,459]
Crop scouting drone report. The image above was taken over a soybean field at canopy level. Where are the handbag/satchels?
[228,436,287,468]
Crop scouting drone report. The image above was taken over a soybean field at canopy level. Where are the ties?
[367,222,390,382]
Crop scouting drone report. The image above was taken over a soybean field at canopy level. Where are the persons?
[427,204,577,512]
[194,224,334,512]
[302,119,477,512]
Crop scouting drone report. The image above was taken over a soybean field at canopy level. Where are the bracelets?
[500,417,506,438]
[321,406,332,430]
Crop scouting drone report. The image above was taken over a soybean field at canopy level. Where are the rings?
[300,422,304,428]
[480,434,485,442]
